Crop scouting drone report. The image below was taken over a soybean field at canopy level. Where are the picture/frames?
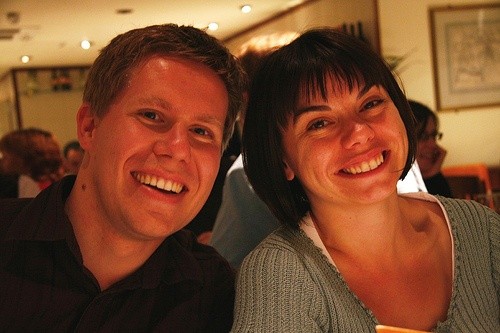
[428,3,500,112]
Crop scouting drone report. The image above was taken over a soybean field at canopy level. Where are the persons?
[232,29,500,333]
[0,24,250,333]
[178,32,303,272]
[406,99,454,198]
[0,127,82,199]
